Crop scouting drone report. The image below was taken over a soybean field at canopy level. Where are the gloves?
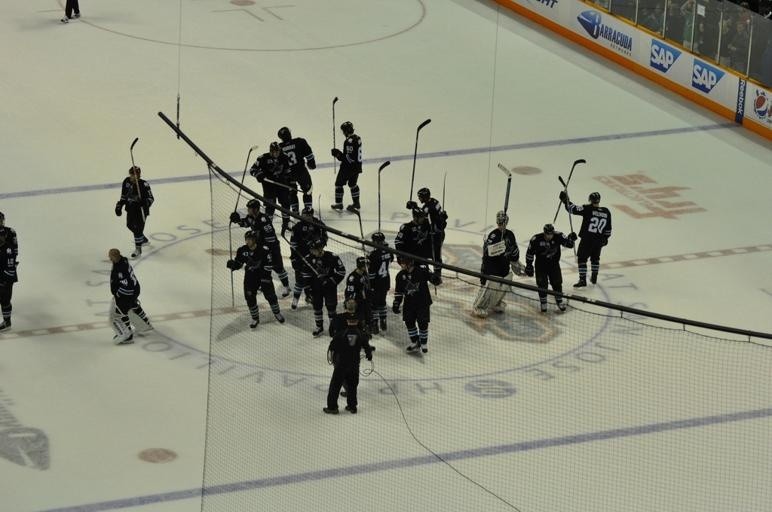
[256,173,266,183]
[332,148,343,161]
[525,265,535,277]
[406,201,418,209]
[559,191,569,204]
[115,201,124,216]
[226,259,242,272]
[568,232,577,242]
[230,212,241,224]
[307,158,316,170]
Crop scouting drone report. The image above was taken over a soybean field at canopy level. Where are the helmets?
[270,142,283,159]
[245,231,258,248]
[340,121,354,138]
[417,188,430,203]
[544,224,554,234]
[129,166,140,183]
[588,192,600,204]
[278,127,291,142]
[302,207,429,327]
[245,200,260,217]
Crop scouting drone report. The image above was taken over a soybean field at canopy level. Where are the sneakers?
[407,341,419,351]
[331,204,343,210]
[556,299,566,311]
[341,392,347,397]
[249,319,259,328]
[0,320,11,329]
[313,327,323,335]
[61,15,69,23]
[345,406,356,413]
[142,238,148,243]
[323,408,338,414]
[132,249,141,257]
[420,343,428,352]
[70,12,80,19]
[274,312,284,323]
[282,284,291,297]
[292,297,298,309]
[541,301,547,312]
[348,203,360,209]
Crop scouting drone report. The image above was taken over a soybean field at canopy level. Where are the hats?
[496,210,509,226]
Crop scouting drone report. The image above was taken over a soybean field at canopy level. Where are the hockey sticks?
[559,176,576,256]
[347,205,368,274]
[410,120,431,201]
[281,222,319,274]
[234,145,258,213]
[130,137,145,222]
[378,161,390,233]
[333,96,338,174]
[264,178,313,195]
[553,159,586,225]
[498,164,511,242]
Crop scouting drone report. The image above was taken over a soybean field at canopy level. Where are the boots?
[573,275,586,287]
[590,271,598,284]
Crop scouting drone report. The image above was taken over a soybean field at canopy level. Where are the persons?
[59,0,80,24]
[114,166,155,260]
[559,190,611,291]
[225,121,450,413]
[108,248,155,346]
[526,224,577,316]
[587,0,772,88]
[0,211,20,334]
[471,210,526,319]
